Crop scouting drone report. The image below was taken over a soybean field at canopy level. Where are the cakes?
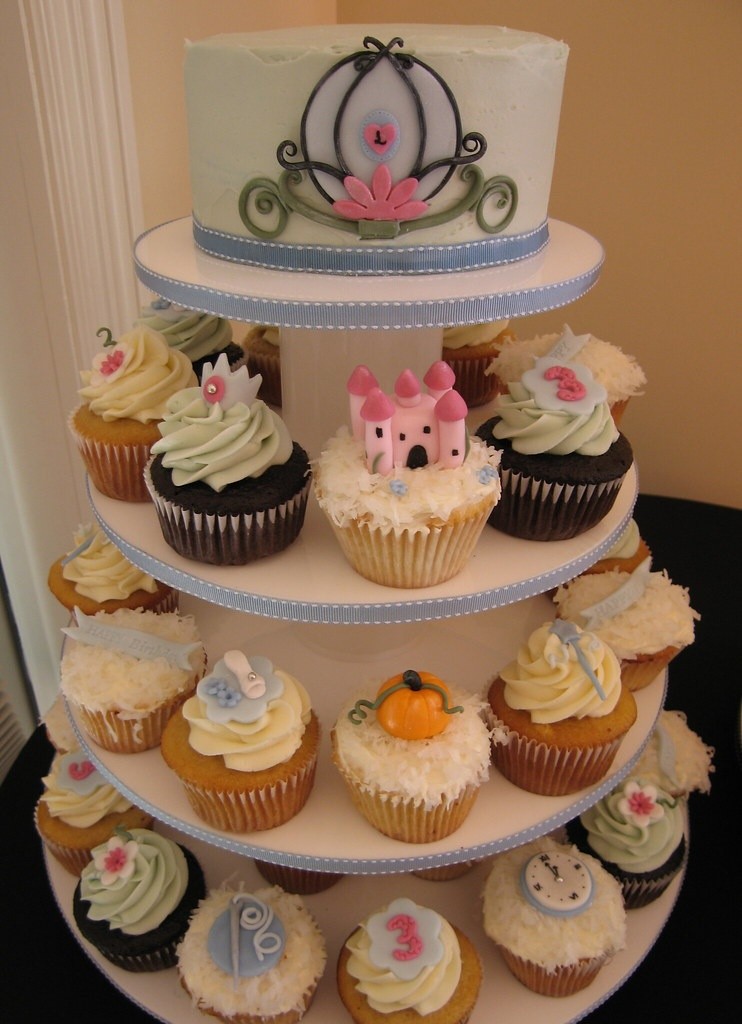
[187,25,567,277]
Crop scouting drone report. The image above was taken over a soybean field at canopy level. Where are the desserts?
[35,297,711,1024]
